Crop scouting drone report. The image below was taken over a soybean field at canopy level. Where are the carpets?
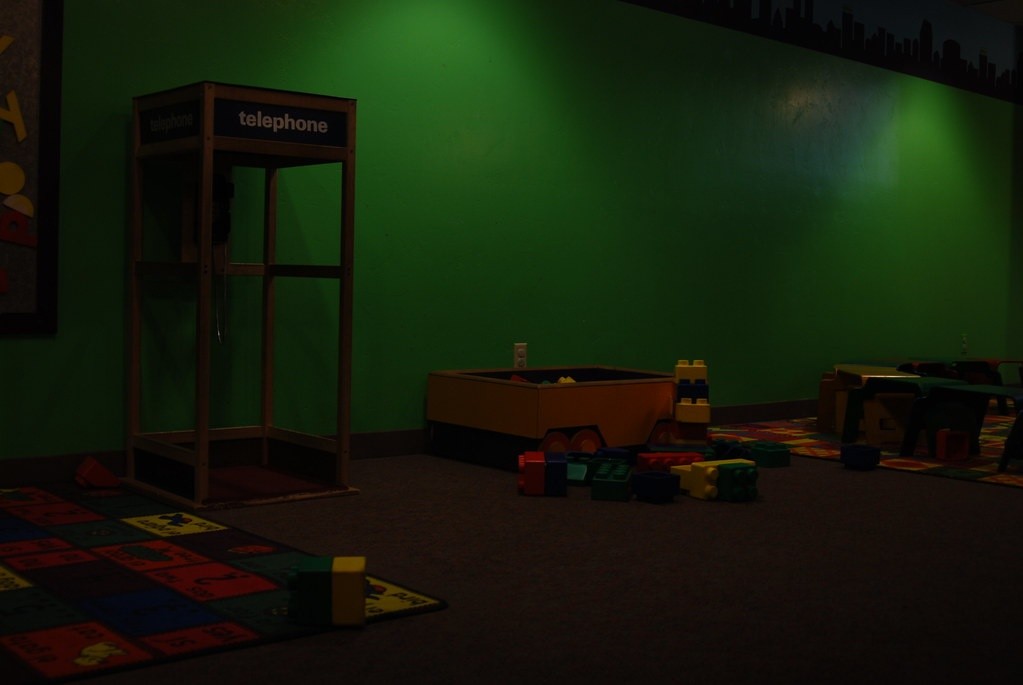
[0,455,443,685]
[707,413,1023,488]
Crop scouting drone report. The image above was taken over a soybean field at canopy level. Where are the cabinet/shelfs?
[115,79,361,514]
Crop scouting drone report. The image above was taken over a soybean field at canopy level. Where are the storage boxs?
[423,365,675,465]
